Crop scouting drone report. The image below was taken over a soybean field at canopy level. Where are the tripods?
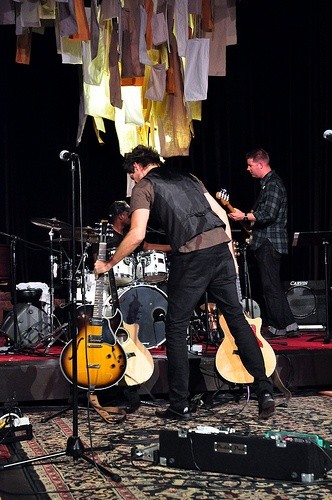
[0,160,123,483]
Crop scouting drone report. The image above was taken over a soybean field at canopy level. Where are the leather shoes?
[155,406,190,421]
[257,391,275,418]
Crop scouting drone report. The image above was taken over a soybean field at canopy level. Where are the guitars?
[216,187,256,246]
[215,240,278,385]
[58,219,127,393]
[105,249,155,387]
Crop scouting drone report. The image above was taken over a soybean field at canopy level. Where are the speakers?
[284,280,327,331]
[1,301,51,348]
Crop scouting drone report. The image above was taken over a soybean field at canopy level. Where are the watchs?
[243,212,248,221]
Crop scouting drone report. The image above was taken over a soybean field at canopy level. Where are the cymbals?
[30,218,66,230]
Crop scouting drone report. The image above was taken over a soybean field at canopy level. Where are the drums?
[107,284,167,351]
[135,249,168,281]
[106,255,135,281]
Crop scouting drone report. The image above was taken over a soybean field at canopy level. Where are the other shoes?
[286,328,300,338]
[262,330,285,340]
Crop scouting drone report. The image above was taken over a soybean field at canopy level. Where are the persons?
[93,205,172,266]
[227,148,304,339]
[93,144,276,419]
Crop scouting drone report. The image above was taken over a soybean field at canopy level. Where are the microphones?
[160,314,166,319]
[60,150,79,161]
[323,130,332,142]
